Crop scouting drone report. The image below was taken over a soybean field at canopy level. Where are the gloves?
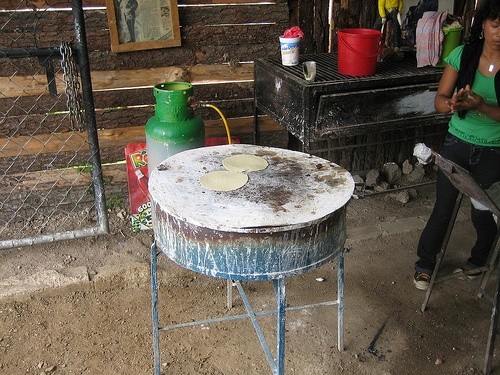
[382,17,386,24]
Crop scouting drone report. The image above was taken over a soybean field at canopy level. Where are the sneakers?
[454,262,484,281]
[413,271,433,290]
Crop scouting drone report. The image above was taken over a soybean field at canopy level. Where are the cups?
[279,35,301,66]
[302,61,316,83]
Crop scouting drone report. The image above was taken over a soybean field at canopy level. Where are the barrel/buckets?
[434,26,464,67]
[336,28,386,76]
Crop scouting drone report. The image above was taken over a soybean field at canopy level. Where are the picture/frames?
[105,0,182,53]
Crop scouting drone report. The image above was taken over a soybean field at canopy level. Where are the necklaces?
[483,52,500,73]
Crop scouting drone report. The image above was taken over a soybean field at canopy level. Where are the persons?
[123,0,138,43]
[413,0,500,290]
[374,0,404,53]
